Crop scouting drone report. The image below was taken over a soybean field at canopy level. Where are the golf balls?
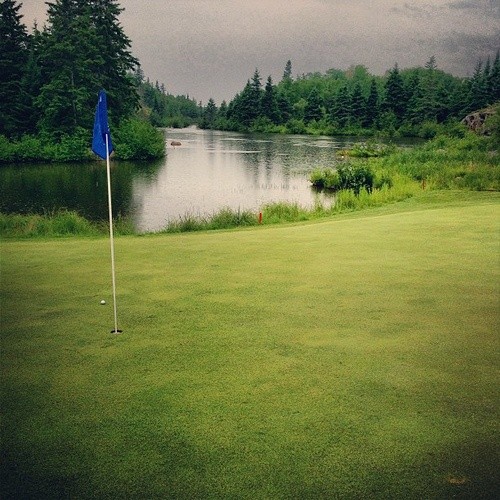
[100,300,106,304]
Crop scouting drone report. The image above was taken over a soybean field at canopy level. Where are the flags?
[90,89,113,158]
